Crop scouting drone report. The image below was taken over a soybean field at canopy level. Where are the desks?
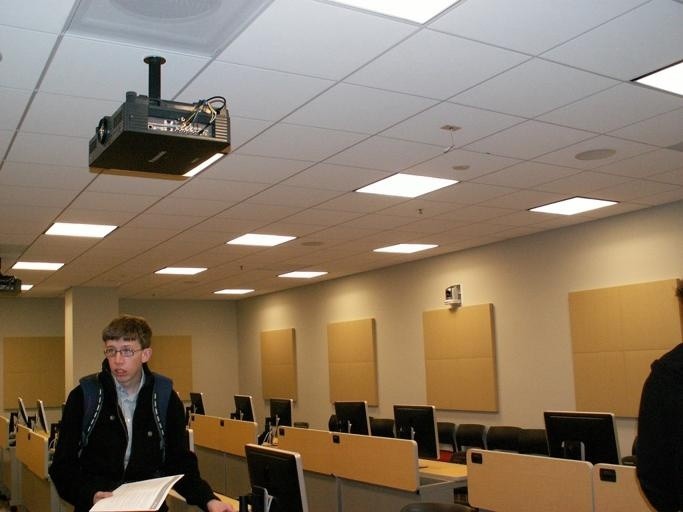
[0,413,657,512]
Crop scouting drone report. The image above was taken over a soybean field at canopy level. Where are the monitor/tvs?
[190,391,205,414]
[18,397,29,424]
[240,443,308,512]
[187,427,197,455]
[392,404,442,462]
[234,394,256,424]
[543,408,619,464]
[269,397,292,427]
[37,399,51,433]
[334,399,371,435]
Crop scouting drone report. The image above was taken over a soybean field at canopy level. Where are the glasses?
[103,346,142,358]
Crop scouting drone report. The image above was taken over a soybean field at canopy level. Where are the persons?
[46,311,238,511]
[634,277,682,511]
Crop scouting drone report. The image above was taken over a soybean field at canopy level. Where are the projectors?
[88,91,232,183]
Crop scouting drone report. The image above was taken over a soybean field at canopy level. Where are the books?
[89,470,183,510]
